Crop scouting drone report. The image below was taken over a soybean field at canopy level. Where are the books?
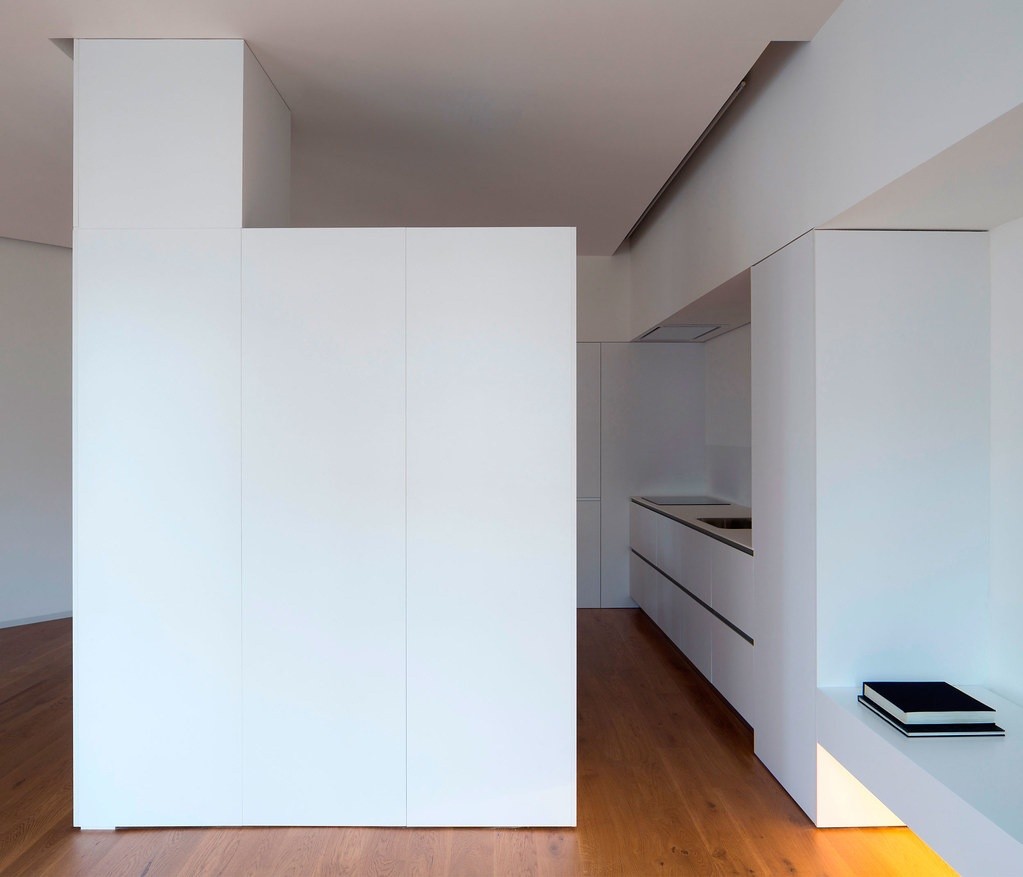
[858,681,1006,738]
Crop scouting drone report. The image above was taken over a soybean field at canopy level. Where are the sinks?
[695,517,752,530]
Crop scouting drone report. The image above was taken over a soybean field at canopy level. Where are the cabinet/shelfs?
[627,502,753,731]
